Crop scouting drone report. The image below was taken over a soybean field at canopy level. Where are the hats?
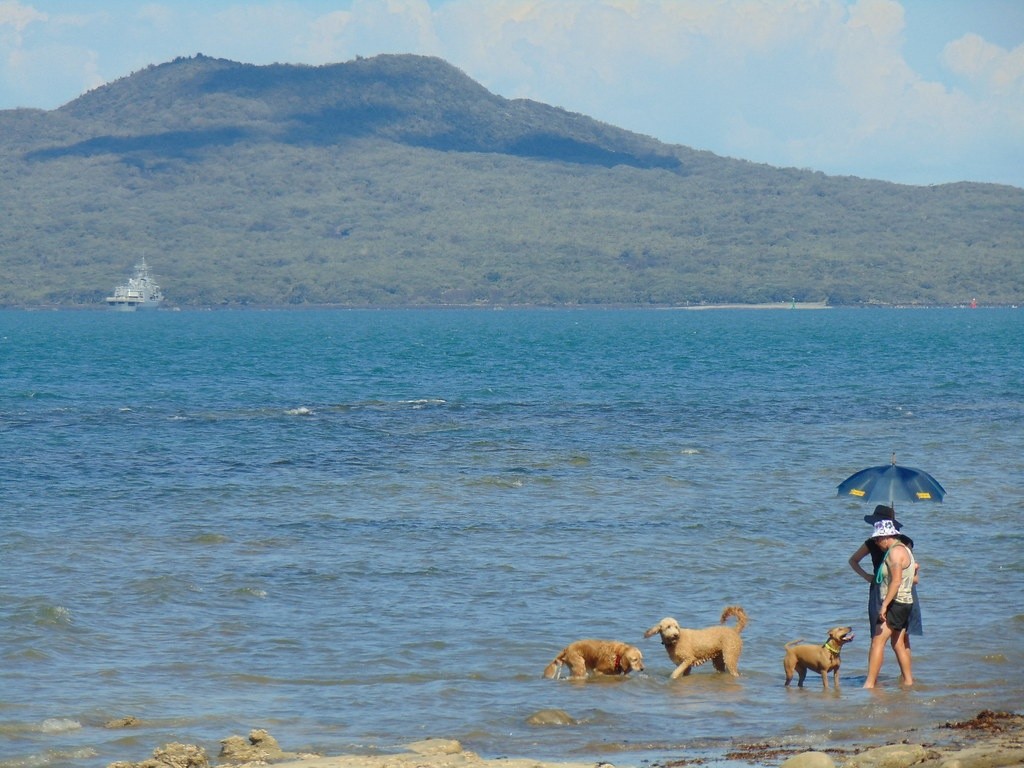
[869,519,901,538]
[864,504,903,532]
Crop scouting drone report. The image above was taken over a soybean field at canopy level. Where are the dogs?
[642,605,749,679]
[783,626,856,688]
[543,638,644,680]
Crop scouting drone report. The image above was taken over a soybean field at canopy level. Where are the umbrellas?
[836,452,948,521]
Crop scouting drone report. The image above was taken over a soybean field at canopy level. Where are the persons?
[850,505,923,660]
[864,519,915,689]
